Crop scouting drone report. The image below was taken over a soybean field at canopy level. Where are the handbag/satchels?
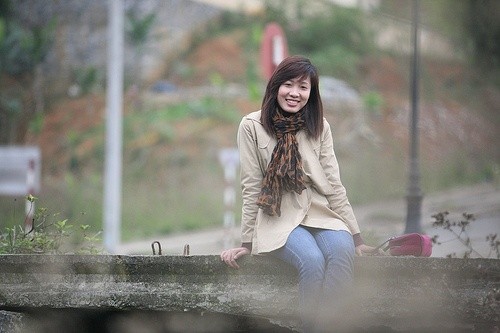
[372,232,433,257]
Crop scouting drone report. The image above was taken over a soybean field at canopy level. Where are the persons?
[218,55,386,330]
